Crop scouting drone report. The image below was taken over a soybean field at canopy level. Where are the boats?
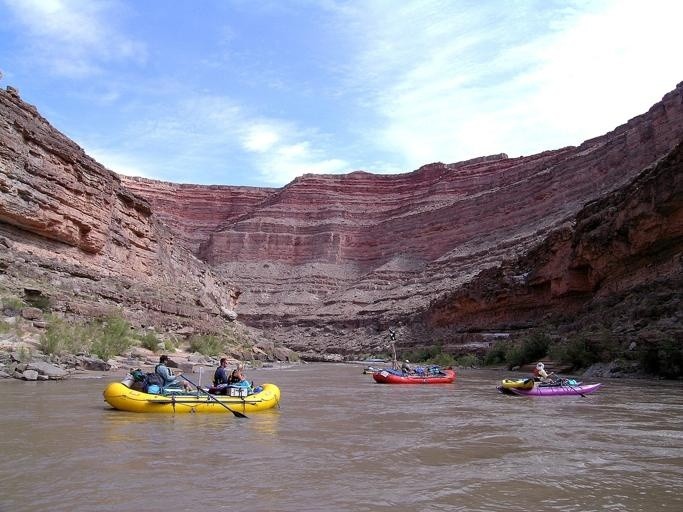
[364,367,452,374]
[103,382,281,414]
[496,378,602,396]
[373,370,456,384]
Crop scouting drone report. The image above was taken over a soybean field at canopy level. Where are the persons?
[229,361,254,394]
[532,361,557,384]
[211,356,228,384]
[154,354,193,392]
[401,359,414,374]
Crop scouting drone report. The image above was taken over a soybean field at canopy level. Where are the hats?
[537,362,544,370]
[160,355,170,361]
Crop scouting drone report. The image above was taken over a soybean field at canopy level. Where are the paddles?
[553,374,587,398]
[180,375,247,418]
[148,400,262,403]
